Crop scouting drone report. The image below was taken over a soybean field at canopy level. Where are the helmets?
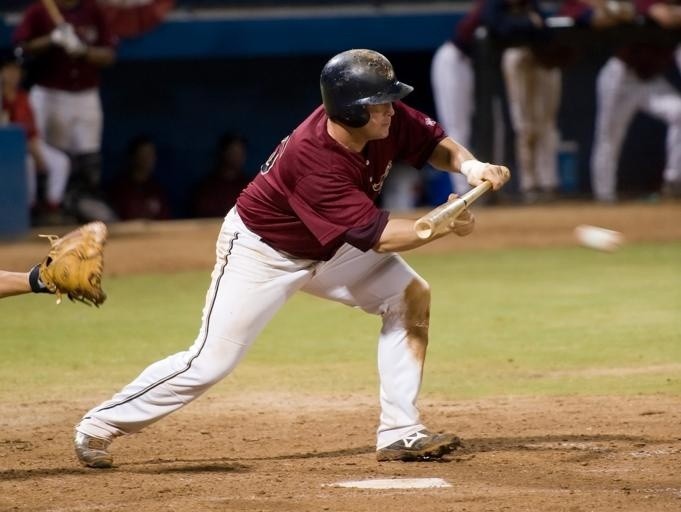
[317,46,414,128]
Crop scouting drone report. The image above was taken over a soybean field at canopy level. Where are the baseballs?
[585,229,611,250]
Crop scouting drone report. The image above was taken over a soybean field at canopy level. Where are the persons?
[105,137,170,218]
[73,51,513,470]
[15,1,121,226]
[0,223,111,307]
[1,41,75,225]
[429,1,680,207]
[182,135,255,219]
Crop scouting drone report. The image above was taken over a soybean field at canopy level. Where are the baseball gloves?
[38,220,108,308]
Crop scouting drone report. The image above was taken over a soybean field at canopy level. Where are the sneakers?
[72,428,114,470]
[375,428,461,463]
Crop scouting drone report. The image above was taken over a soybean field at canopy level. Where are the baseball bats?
[414,179,492,241]
